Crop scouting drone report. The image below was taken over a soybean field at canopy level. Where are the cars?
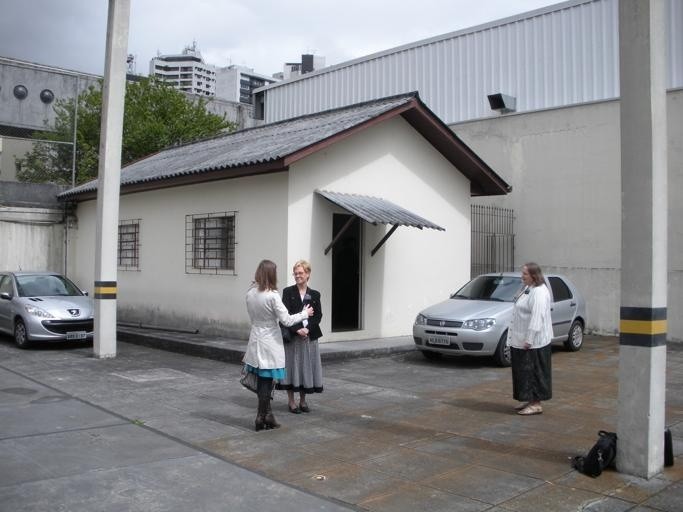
[410,269,587,366]
[0,269,96,351]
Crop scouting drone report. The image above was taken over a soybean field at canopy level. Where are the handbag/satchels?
[240,373,275,399]
[585,430,617,476]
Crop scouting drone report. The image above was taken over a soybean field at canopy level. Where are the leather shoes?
[287,400,308,414]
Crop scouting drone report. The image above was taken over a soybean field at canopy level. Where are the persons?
[236,258,315,433]
[278,260,326,415]
[504,261,555,416]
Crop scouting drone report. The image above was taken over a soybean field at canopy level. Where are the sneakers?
[514,401,543,415]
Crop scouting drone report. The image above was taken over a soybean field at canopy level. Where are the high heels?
[255,413,279,430]
